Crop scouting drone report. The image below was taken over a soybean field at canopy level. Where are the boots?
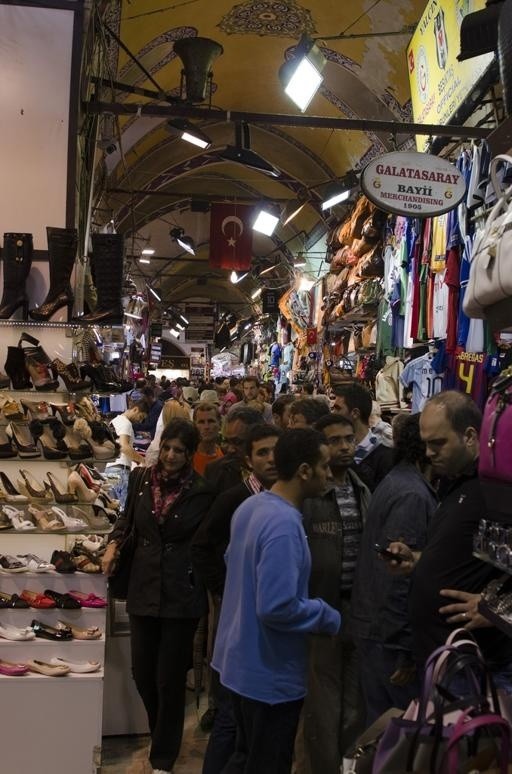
[29,227,78,324]
[76,233,124,324]
[85,273,98,311]
[1,233,33,321]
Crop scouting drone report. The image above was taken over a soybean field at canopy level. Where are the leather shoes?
[0,620,36,640]
[51,656,101,672]
[26,656,71,675]
[1,659,30,675]
[31,619,72,640]
[44,589,82,609]
[68,590,107,607]
[55,619,102,640]
[1,592,29,608]
[20,589,56,608]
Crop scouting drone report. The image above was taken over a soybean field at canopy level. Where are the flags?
[207,203,253,272]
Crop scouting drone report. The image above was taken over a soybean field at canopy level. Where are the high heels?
[0,394,23,419]
[51,418,93,459]
[25,357,59,391]
[19,332,52,368]
[92,503,119,523]
[17,468,54,504]
[20,398,57,421]
[68,471,95,503]
[4,347,33,389]
[6,421,41,459]
[104,361,133,393]
[51,358,92,392]
[29,419,69,461]
[48,401,76,427]
[0,471,28,504]
[71,505,112,529]
[92,361,123,392]
[28,503,67,531]
[43,472,73,503]
[0,373,11,388]
[0,505,37,531]
[99,491,122,508]
[0,434,18,459]
[80,467,100,491]
[77,463,102,487]
[51,506,88,531]
[74,418,114,459]
[88,419,121,449]
[97,495,118,514]
[80,364,118,391]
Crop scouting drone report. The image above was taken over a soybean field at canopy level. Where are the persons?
[144,409,165,467]
[131,374,331,427]
[376,390,482,686]
[101,416,204,774]
[437,588,512,632]
[332,384,377,465]
[337,411,436,749]
[191,404,224,477]
[204,429,281,655]
[104,401,149,509]
[204,406,265,513]
[201,428,341,774]
[302,413,372,774]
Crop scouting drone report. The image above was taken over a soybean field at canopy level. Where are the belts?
[110,464,131,471]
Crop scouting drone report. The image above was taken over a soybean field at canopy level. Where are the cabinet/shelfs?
[0,567,109,774]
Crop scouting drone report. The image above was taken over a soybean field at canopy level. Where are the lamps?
[145,283,162,302]
[258,260,276,277]
[279,55,325,115]
[139,255,151,264]
[164,119,211,149]
[320,187,351,211]
[142,243,156,255]
[177,238,195,256]
[236,272,248,282]
[220,145,281,177]
[251,209,280,237]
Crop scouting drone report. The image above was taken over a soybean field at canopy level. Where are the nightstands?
[0,313,125,534]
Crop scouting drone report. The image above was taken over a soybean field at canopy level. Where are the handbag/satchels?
[340,631,511,773]
[462,154,512,319]
[478,366,512,476]
[105,467,150,601]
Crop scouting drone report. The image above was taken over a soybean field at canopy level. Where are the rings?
[461,612,466,621]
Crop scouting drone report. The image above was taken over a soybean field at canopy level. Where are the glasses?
[327,436,357,446]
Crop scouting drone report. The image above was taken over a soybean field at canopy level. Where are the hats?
[183,386,199,402]
[194,390,220,403]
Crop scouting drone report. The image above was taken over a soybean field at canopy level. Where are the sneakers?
[1,553,29,573]
[186,668,206,692]
[152,769,172,774]
[70,550,102,573]
[51,550,76,574]
[16,554,56,572]
[73,543,101,565]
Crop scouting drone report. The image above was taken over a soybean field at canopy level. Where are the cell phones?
[374,542,400,559]
